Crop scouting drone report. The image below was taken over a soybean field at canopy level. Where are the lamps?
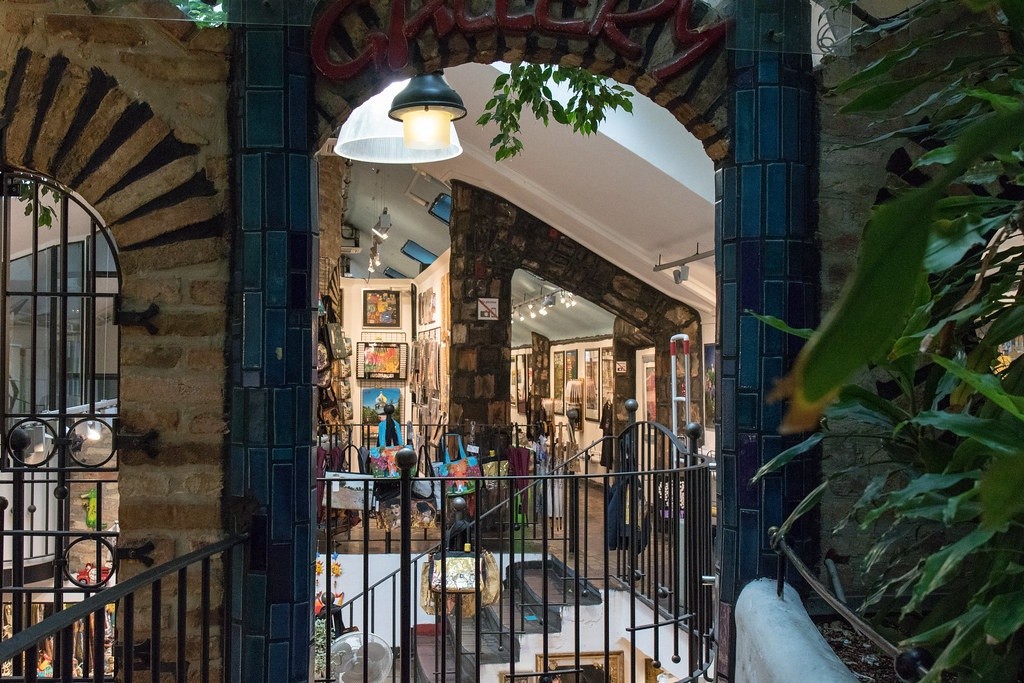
[518,305,525,321]
[333,79,463,163]
[539,295,556,316]
[388,69,467,150]
[673,264,689,284]
[368,236,382,273]
[560,289,576,308]
[527,301,537,318]
[371,220,392,239]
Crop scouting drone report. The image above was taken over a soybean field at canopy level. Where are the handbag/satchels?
[420,520,504,619]
[318,294,353,446]
[322,420,581,531]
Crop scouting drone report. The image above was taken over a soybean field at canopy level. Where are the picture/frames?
[527,354,533,400]
[516,354,526,414]
[553,351,565,416]
[584,347,600,422]
[566,349,578,415]
[642,354,655,441]
[535,650,625,683]
[362,288,402,329]
[498,670,534,683]
[703,343,716,428]
[601,347,613,412]
